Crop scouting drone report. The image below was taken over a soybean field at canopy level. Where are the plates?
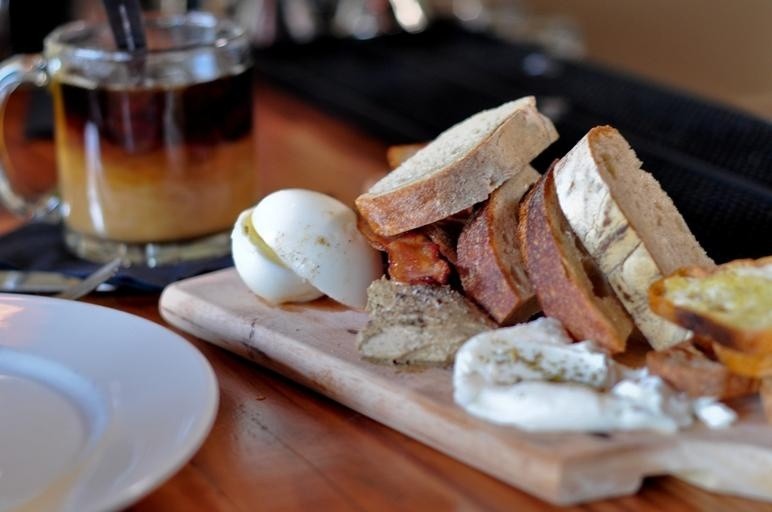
[0,293,221,512]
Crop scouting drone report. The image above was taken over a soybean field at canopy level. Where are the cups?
[0,9,260,270]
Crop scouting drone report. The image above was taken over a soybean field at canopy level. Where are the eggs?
[229,187,382,310]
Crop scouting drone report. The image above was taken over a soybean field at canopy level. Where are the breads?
[356,93,772,435]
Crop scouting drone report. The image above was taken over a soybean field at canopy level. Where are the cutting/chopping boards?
[158,265,772,507]
